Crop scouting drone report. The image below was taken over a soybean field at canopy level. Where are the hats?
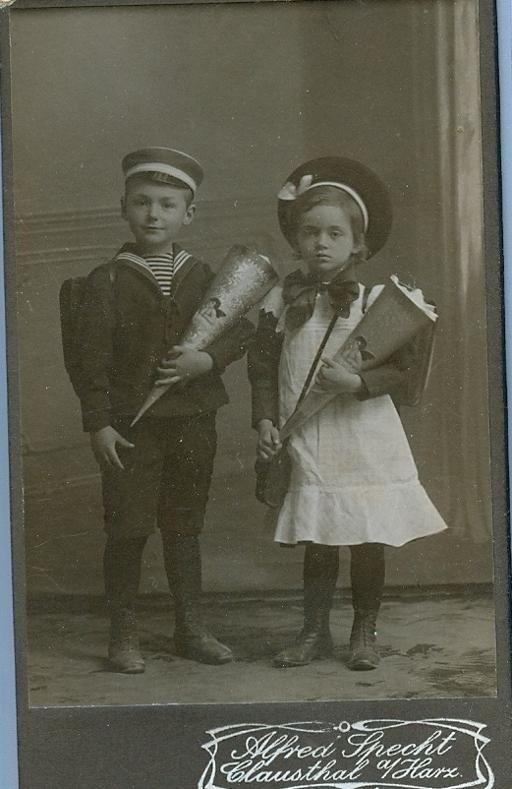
[277,156,393,261]
[122,147,205,202]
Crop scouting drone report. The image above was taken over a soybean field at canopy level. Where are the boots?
[347,543,386,670]
[103,535,148,675]
[161,533,233,665]
[272,543,340,668]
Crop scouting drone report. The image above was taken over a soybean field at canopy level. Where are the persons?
[57,146,281,675]
[247,156,450,671]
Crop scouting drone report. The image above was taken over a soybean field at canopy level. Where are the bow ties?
[280,267,357,332]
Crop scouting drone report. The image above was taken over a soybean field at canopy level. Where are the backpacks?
[59,263,118,401]
[361,284,437,408]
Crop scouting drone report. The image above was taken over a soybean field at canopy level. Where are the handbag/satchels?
[254,448,291,508]
